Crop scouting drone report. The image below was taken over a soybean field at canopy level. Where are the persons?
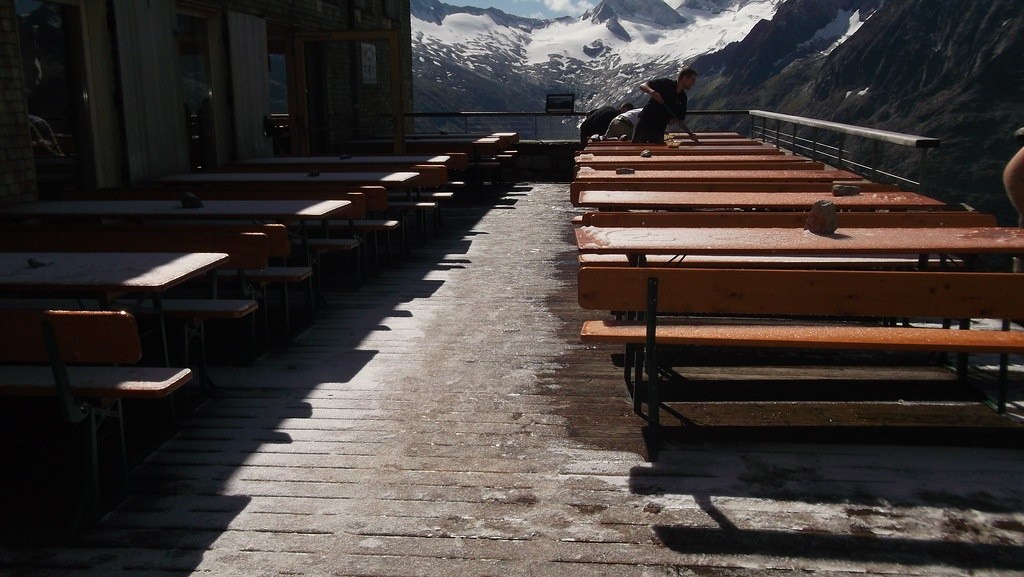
[604,107,675,140]
[1002,144,1024,218]
[580,101,634,149]
[632,67,698,144]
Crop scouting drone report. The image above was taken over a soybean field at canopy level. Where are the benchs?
[571,132,1024,461]
[0,145,517,522]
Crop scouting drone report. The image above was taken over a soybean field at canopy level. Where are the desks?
[576,154,808,162]
[672,138,758,145]
[579,190,945,211]
[576,167,858,179]
[580,146,783,152]
[575,226,1024,412]
[0,197,353,347]
[228,156,451,232]
[0,253,229,463]
[407,133,520,141]
[132,172,421,275]
[664,131,739,137]
[405,137,500,157]
